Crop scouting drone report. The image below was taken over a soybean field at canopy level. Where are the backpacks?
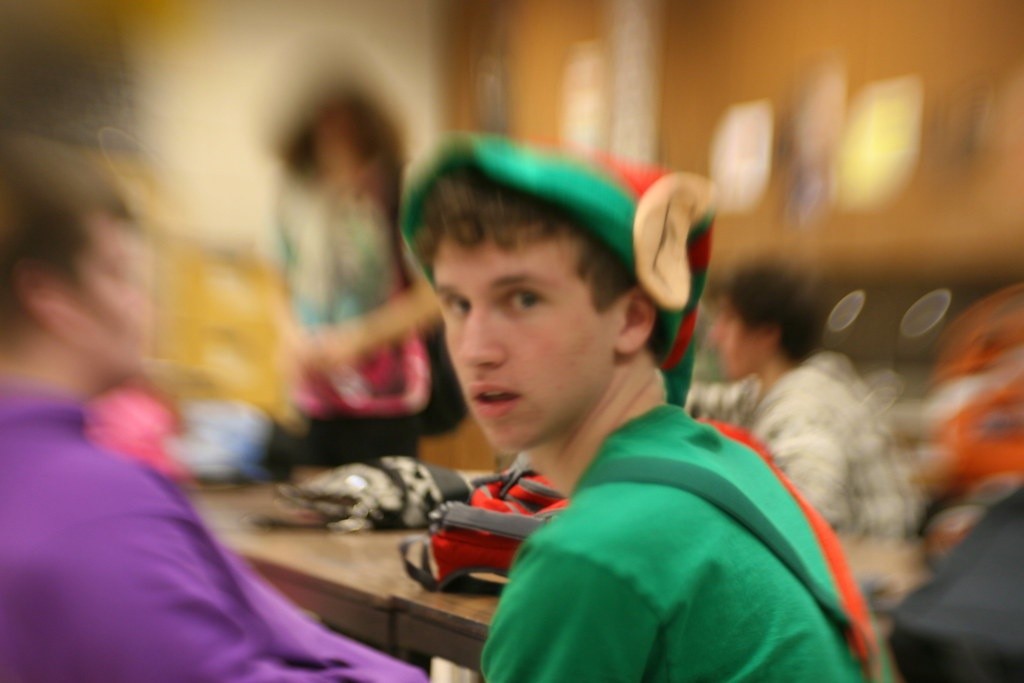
[400,451,571,591]
[272,449,509,537]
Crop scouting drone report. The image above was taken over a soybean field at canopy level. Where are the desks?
[180,461,509,668]
[387,581,502,683]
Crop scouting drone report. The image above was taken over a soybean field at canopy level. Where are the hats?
[397,132,717,405]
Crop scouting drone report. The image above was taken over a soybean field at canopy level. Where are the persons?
[397,132,896,683]
[0,130,428,683]
[260,79,443,469]
[708,261,924,539]
